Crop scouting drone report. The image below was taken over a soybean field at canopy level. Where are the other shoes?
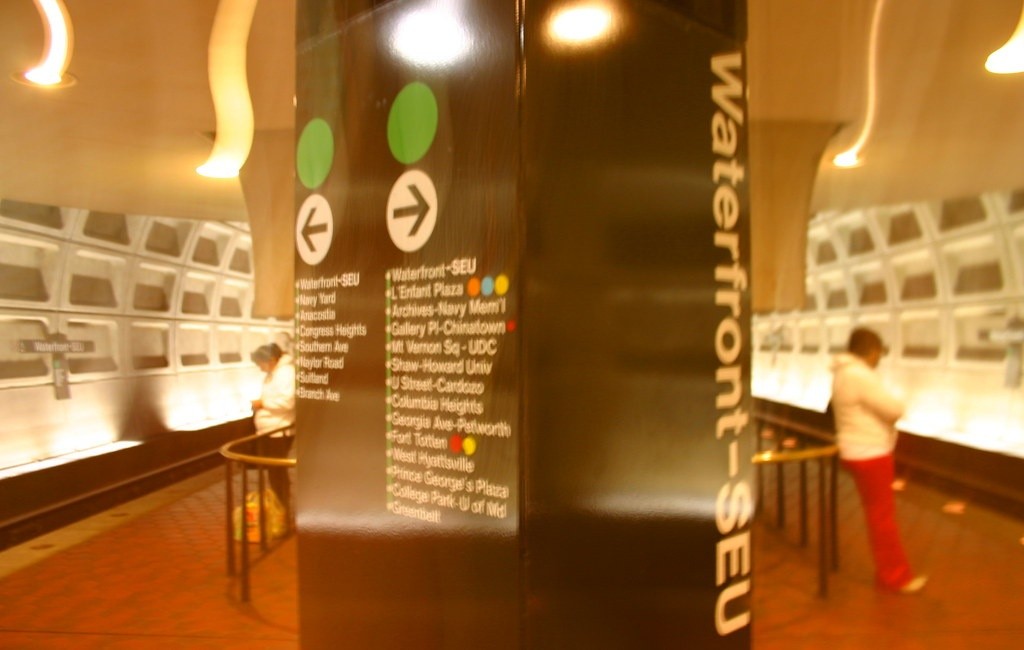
[899,577,928,593]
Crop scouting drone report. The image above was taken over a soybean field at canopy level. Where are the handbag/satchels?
[230,487,287,544]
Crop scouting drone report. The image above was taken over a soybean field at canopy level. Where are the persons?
[828,325,930,593]
[249,343,298,539]
